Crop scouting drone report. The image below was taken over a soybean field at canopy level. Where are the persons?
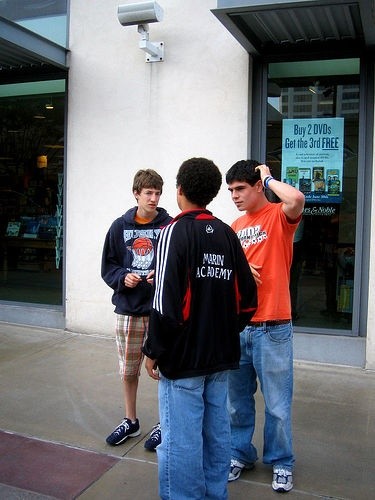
[101,168,174,451]
[226,159,306,491]
[140,158,258,500]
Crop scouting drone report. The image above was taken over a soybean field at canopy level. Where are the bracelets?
[264,176,273,188]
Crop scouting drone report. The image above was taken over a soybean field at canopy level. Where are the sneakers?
[106,417,141,446]
[228,458,257,481]
[271,465,294,492]
[143,422,161,451]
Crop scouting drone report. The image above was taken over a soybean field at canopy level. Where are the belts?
[245,319,291,329]
